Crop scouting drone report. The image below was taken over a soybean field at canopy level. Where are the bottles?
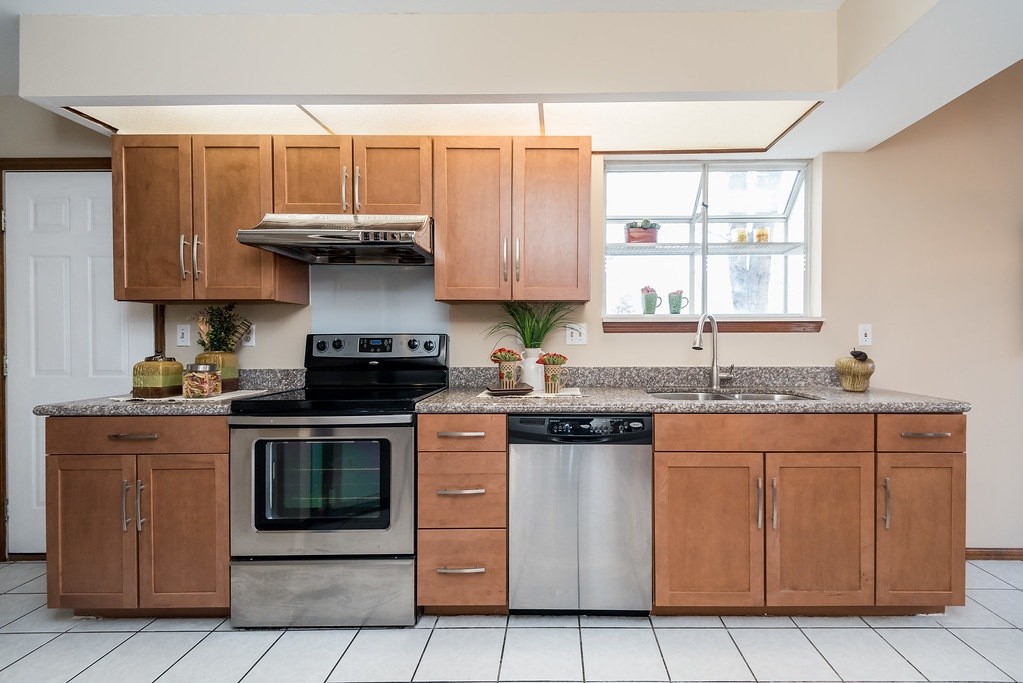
[181,363,223,398]
[132,355,184,398]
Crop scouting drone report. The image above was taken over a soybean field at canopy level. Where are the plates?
[486,383,534,396]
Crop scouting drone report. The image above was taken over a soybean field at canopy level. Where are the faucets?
[691,313,734,388]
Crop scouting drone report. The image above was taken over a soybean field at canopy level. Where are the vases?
[668,293,689,315]
[498,361,518,389]
[544,364,562,394]
[640,293,662,315]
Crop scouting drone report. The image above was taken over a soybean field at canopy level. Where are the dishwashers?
[508,414,655,617]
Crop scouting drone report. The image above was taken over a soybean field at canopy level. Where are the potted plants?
[624,219,661,243]
[479,303,586,390]
[187,305,246,393]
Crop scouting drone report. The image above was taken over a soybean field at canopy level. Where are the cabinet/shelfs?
[416,413,510,616]
[112,133,594,305]
[653,412,967,616]
[45,415,231,618]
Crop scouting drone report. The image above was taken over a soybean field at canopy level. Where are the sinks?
[643,387,733,401]
[720,390,827,400]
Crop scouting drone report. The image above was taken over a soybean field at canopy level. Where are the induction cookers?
[232,333,450,412]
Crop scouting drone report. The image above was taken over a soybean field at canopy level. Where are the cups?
[753,226,771,242]
[731,227,749,242]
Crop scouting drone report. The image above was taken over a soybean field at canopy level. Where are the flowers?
[672,290,684,295]
[536,352,569,365]
[640,286,656,294]
[490,348,522,363]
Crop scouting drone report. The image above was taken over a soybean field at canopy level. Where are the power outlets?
[242,325,256,347]
[858,324,872,346]
[565,323,587,345]
[177,325,190,347]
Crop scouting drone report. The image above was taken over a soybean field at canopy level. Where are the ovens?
[227,414,419,629]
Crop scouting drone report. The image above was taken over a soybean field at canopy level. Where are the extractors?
[236,213,435,266]
